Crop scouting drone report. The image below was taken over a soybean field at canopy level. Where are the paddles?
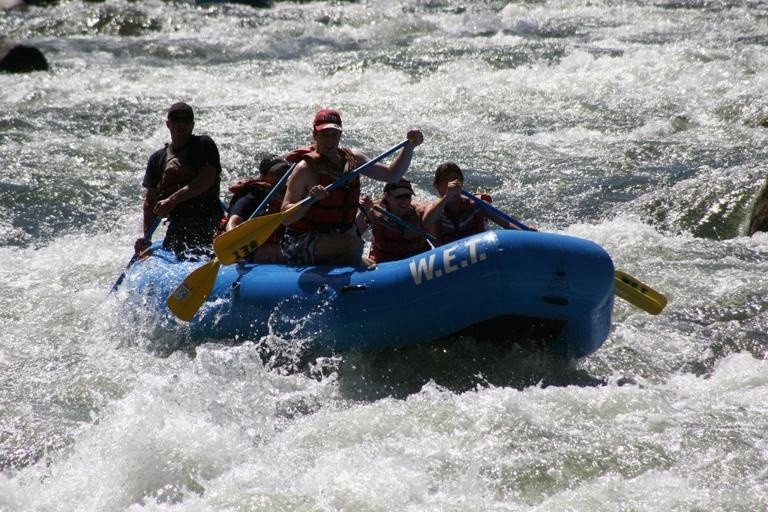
[213,139,410,265]
[461,189,667,315]
[167,163,300,322]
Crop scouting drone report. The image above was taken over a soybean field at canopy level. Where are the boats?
[122,230,616,365]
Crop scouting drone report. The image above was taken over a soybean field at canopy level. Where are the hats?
[383,178,417,198]
[167,102,194,119]
[259,155,291,175]
[313,110,343,133]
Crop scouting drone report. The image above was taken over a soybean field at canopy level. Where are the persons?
[225,155,292,264]
[422,163,538,248]
[134,103,225,256]
[356,177,432,265]
[280,109,424,267]
